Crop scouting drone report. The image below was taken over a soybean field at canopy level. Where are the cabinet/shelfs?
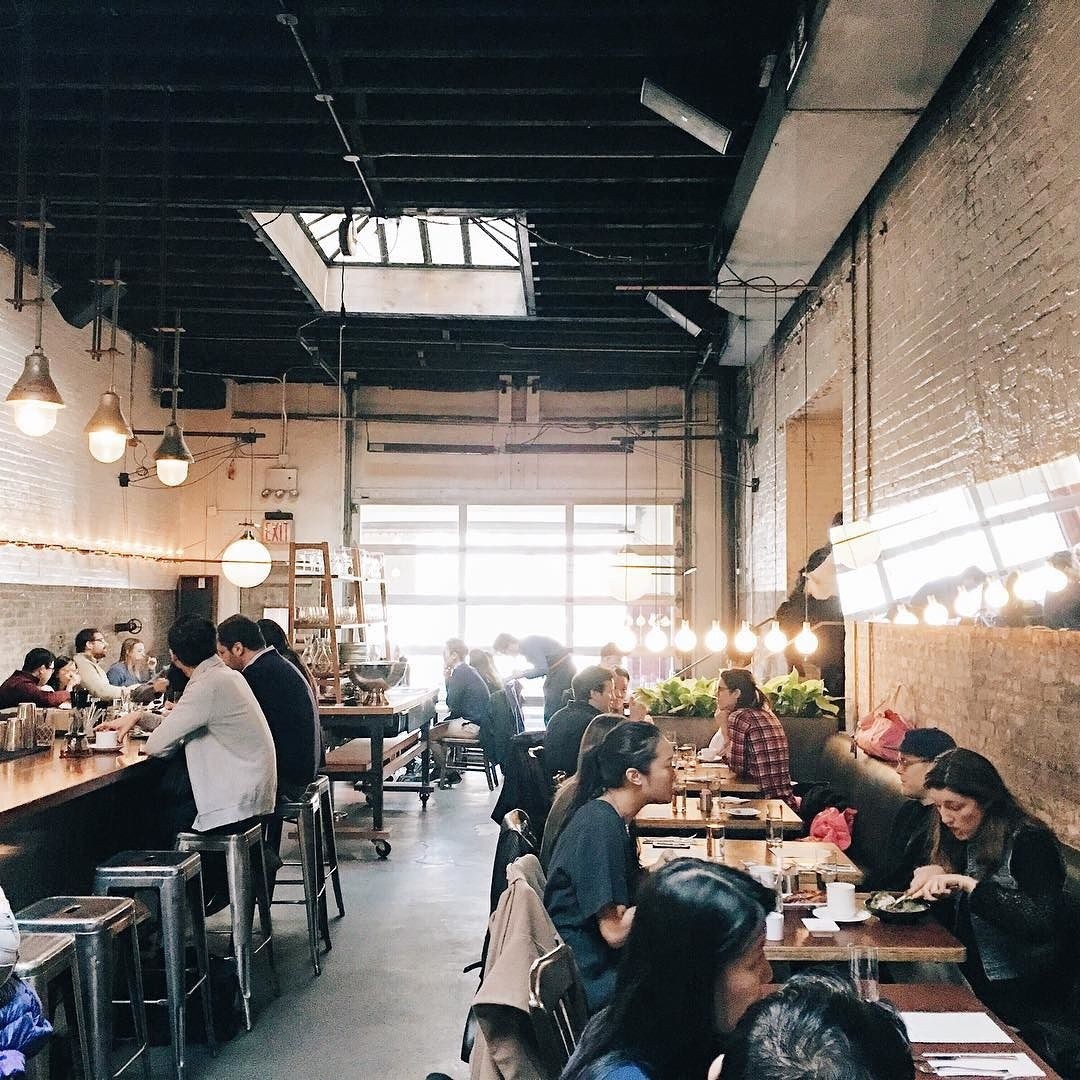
[288,541,392,706]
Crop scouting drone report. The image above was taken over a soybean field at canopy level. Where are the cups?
[848,943,881,1004]
[296,545,382,624]
[174,691,183,703]
[705,804,784,863]
[751,865,786,941]
[113,698,121,712]
[0,702,55,751]
[95,729,120,746]
[673,742,721,815]
[816,846,856,918]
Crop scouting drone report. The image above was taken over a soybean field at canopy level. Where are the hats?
[600,642,630,656]
[881,727,956,756]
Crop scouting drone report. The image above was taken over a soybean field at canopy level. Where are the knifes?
[914,1054,1017,1060]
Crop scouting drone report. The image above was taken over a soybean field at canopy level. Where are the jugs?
[61,733,91,756]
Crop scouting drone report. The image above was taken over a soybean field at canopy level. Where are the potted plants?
[632,665,854,780]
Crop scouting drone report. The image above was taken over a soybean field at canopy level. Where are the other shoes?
[444,771,462,784]
[430,767,442,781]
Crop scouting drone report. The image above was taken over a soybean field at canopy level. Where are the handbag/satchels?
[800,785,849,823]
[850,699,915,761]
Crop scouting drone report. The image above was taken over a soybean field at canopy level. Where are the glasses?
[90,638,106,643]
[898,758,932,769]
[714,686,732,694]
[45,667,55,673]
[59,668,80,675]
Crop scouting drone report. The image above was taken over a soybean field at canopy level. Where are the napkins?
[897,1011,1014,1044]
[922,1051,1047,1077]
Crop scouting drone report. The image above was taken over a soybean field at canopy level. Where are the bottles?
[301,633,335,677]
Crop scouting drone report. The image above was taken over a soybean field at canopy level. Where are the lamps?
[6,195,65,438]
[132,429,271,588]
[153,308,195,487]
[81,260,134,462]
[611,285,818,656]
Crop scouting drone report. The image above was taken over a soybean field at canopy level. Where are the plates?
[697,756,721,762]
[340,642,369,668]
[726,807,761,816]
[90,744,123,752]
[814,907,872,922]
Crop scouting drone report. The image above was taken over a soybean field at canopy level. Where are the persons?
[106,638,157,687]
[707,963,914,1080]
[904,748,1070,1026]
[0,647,71,710]
[492,634,576,726]
[425,638,503,785]
[540,644,652,879]
[559,856,777,1080]
[41,655,114,710]
[858,729,959,894]
[542,720,677,1022]
[92,612,325,916]
[72,628,141,703]
[714,668,799,816]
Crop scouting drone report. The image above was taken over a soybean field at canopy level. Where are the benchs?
[822,733,1080,1080]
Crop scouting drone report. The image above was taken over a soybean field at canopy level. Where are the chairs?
[439,680,526,791]
[427,731,589,1080]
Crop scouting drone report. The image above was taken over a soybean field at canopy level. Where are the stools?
[15,895,152,1080]
[158,822,280,1032]
[13,931,91,1080]
[94,850,218,1080]
[230,775,344,975]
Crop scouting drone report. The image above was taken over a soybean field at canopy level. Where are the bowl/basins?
[865,893,931,925]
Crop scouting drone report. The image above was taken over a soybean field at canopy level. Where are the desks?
[637,837,864,885]
[670,752,762,792]
[633,798,803,830]
[760,891,967,962]
[758,984,1064,1080]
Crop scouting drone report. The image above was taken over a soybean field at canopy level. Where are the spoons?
[914,1063,1008,1073]
